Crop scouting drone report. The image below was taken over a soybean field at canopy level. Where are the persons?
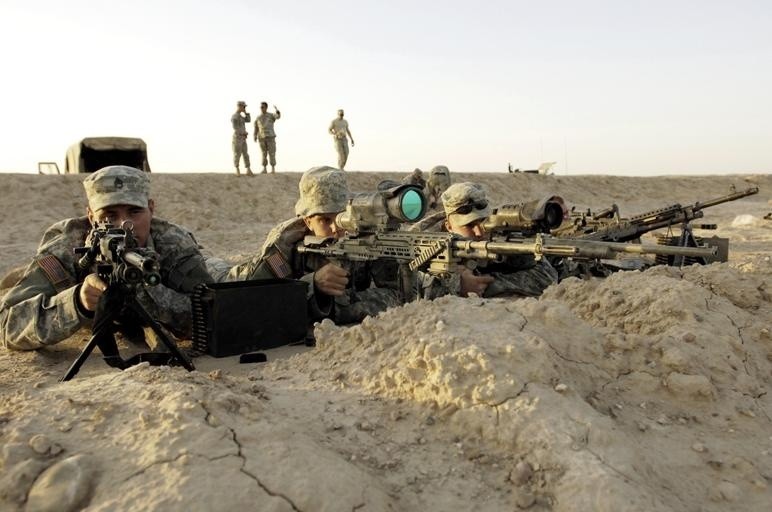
[1,164,222,351]
[328,109,355,171]
[197,167,407,330]
[408,181,558,303]
[230,100,255,177]
[544,195,581,282]
[253,102,282,173]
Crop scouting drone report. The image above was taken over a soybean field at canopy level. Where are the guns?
[295,185,615,306]
[450,194,718,284]
[552,186,760,268]
[62,219,197,382]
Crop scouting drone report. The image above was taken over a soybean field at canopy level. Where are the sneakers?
[245,168,254,176]
[235,168,243,177]
[261,169,275,174]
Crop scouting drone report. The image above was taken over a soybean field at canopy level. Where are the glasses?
[445,198,489,222]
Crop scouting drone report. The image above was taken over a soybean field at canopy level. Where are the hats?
[82,165,151,213]
[439,181,491,227]
[412,168,422,180]
[337,109,345,117]
[294,166,353,220]
[430,165,450,186]
[237,101,247,106]
